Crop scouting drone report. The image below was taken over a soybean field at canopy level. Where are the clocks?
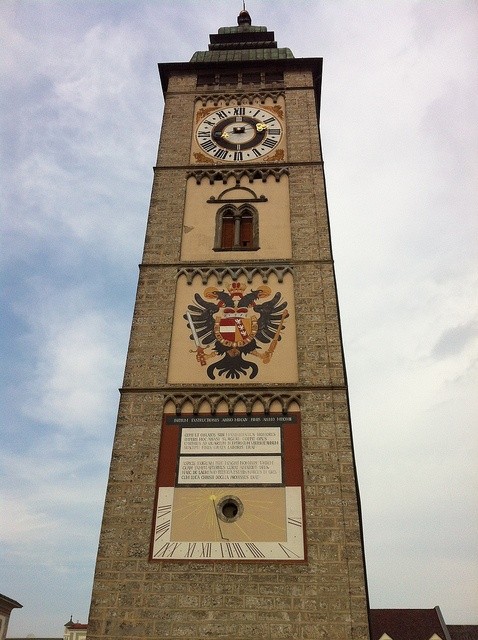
[196,106,283,163]
